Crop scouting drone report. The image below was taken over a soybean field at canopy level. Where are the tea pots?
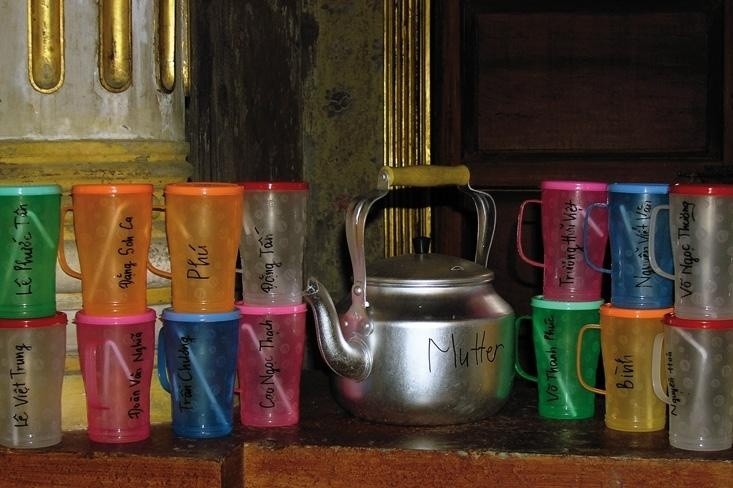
[304,165,520,429]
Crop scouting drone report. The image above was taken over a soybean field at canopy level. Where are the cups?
[74,307,156,445]
[648,183,732,322]
[237,182,302,304]
[583,184,673,311]
[578,302,673,433]
[1,310,68,450]
[147,183,246,315]
[158,306,240,438]
[651,312,732,453]
[57,184,155,317]
[235,299,307,430]
[1,184,64,318]
[516,182,609,302]
[515,295,604,421]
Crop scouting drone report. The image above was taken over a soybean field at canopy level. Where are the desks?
[0,377,733,488]
